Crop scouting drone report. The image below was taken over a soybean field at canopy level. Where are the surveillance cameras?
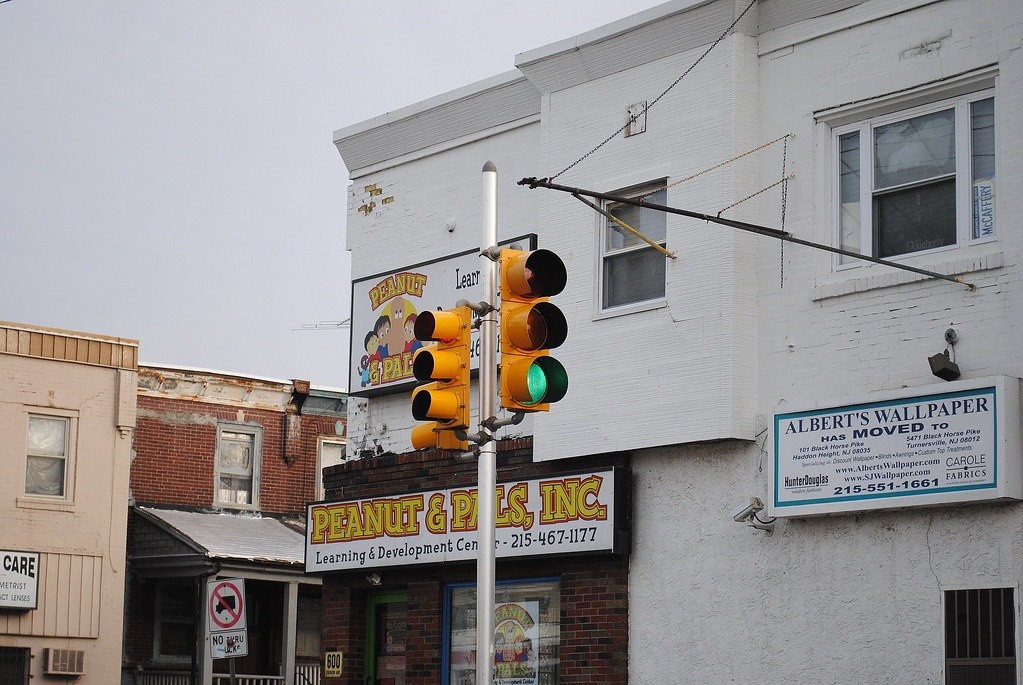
[732,497,764,522]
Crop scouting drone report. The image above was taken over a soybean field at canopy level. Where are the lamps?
[928,353,960,381]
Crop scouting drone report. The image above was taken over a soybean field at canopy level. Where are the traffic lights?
[412,306,471,432]
[498,247,569,413]
[410,422,469,452]
